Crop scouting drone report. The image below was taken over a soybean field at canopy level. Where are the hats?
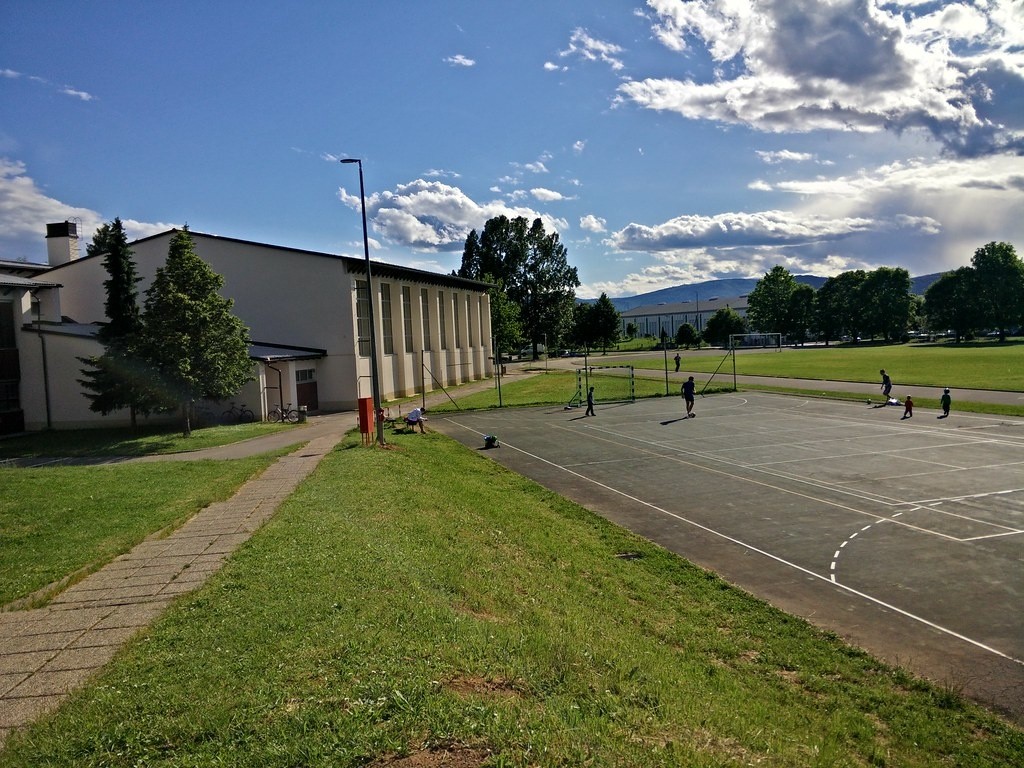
[944,388,950,394]
[906,395,912,399]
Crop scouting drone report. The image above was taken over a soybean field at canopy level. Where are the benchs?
[385,415,397,428]
[403,418,418,432]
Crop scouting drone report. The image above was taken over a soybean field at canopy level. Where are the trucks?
[521,343,545,356]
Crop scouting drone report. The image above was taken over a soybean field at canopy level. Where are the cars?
[907,330,956,340]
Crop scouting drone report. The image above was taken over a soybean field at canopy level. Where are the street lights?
[340,158,380,411]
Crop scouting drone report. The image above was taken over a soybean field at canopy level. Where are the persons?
[674,353,681,372]
[903,394,913,417]
[585,387,596,416]
[408,407,428,433]
[681,376,696,417]
[940,388,951,415]
[879,369,894,405]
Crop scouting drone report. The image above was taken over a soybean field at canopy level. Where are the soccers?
[690,412,696,418]
[867,399,871,404]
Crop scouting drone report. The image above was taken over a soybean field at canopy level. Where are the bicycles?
[220,402,255,423]
[267,403,299,423]
